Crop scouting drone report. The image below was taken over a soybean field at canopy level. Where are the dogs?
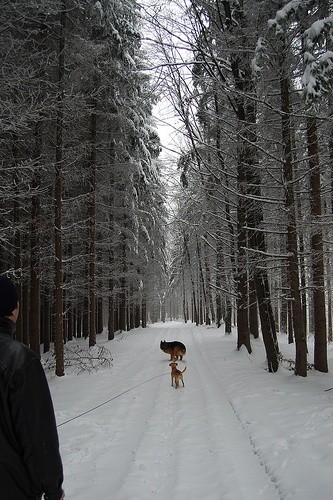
[160,340,186,361]
[169,363,186,389]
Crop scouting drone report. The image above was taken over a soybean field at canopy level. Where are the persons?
[0,274,67,500]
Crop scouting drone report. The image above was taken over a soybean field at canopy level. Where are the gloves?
[44,491,65,500]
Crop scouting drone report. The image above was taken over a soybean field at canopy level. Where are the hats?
[0,275,18,316]
